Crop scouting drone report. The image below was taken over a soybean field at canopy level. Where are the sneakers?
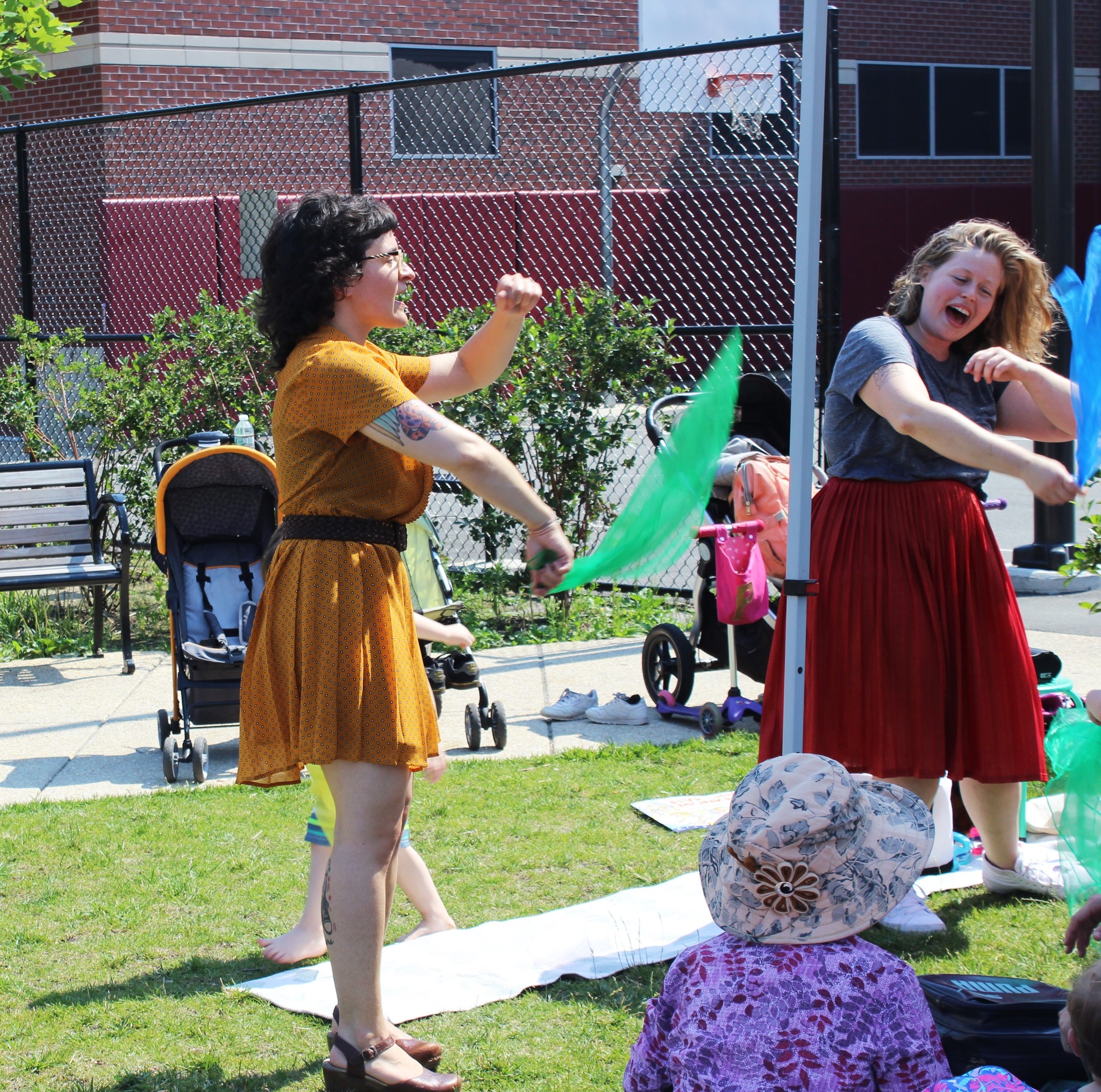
[1041,694,1086,732]
[585,692,648,726]
[540,688,598,722]
[982,850,1065,899]
[423,655,445,695]
[323,1033,461,1092]
[436,651,479,686]
[881,886,946,932]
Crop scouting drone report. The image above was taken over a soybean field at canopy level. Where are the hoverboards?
[649,519,771,741]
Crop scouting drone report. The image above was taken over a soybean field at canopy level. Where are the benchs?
[0,458,136,674]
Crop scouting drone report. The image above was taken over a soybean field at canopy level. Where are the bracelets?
[527,516,561,536]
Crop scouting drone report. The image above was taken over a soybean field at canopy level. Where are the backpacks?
[728,456,820,580]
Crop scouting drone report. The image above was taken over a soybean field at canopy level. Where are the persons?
[756,216,1080,936]
[233,192,576,1092]
[1055,688,1101,1092]
[621,752,951,1092]
[255,605,477,964]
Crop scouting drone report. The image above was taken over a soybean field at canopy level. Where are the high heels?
[327,1006,442,1068]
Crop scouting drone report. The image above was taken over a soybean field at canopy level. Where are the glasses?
[357,250,404,276]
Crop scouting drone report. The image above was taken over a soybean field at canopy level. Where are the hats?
[698,753,935,944]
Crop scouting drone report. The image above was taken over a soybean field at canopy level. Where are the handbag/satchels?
[918,974,1094,1091]
[714,522,769,623]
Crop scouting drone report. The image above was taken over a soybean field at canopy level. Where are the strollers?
[150,429,291,783]
[398,505,507,754]
[641,371,829,730]
